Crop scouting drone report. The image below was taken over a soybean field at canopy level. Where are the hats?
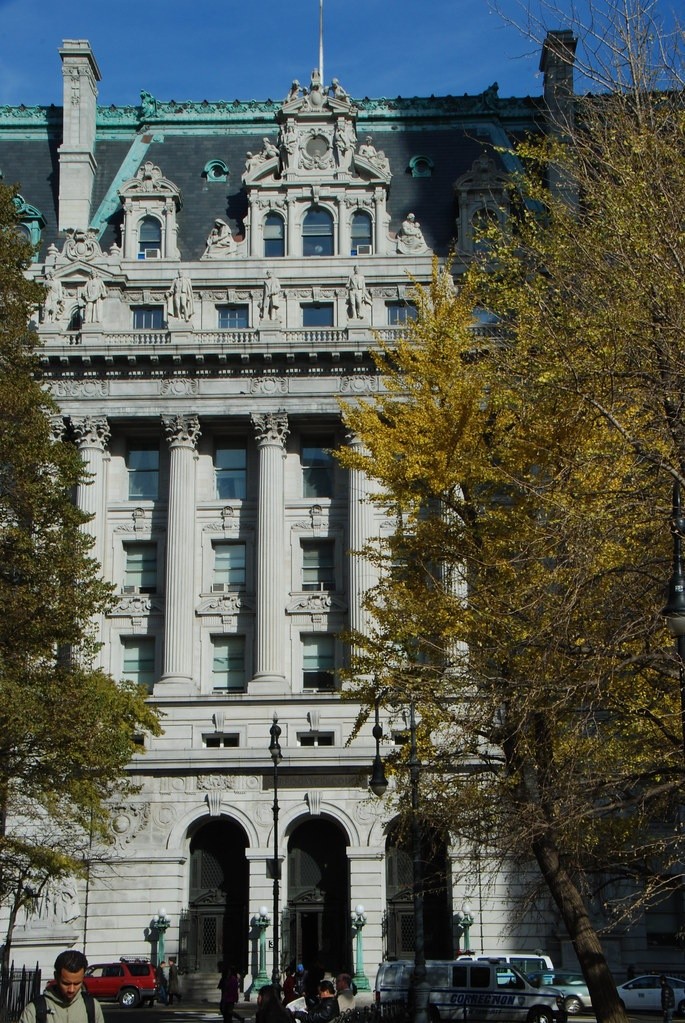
[297,963,304,971]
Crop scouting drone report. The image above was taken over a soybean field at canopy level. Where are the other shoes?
[166,1003,172,1006]
[241,1018,245,1023]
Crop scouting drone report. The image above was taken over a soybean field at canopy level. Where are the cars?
[617,975,685,1018]
[528,970,594,1017]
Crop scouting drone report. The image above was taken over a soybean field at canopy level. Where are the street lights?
[371,683,431,1023]
[268,712,283,987]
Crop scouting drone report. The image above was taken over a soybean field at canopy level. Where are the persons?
[660,975,676,1023]
[335,126,393,177]
[19,950,104,1023]
[397,213,425,254]
[260,269,282,320]
[283,68,350,105]
[166,268,195,323]
[345,264,367,320]
[244,126,297,173]
[206,218,234,249]
[81,268,107,324]
[148,958,182,1008]
[40,270,63,323]
[218,953,358,1023]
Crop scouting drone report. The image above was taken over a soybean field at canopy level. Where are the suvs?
[46,959,160,1010]
[375,960,569,1022]
[445,948,558,985]
